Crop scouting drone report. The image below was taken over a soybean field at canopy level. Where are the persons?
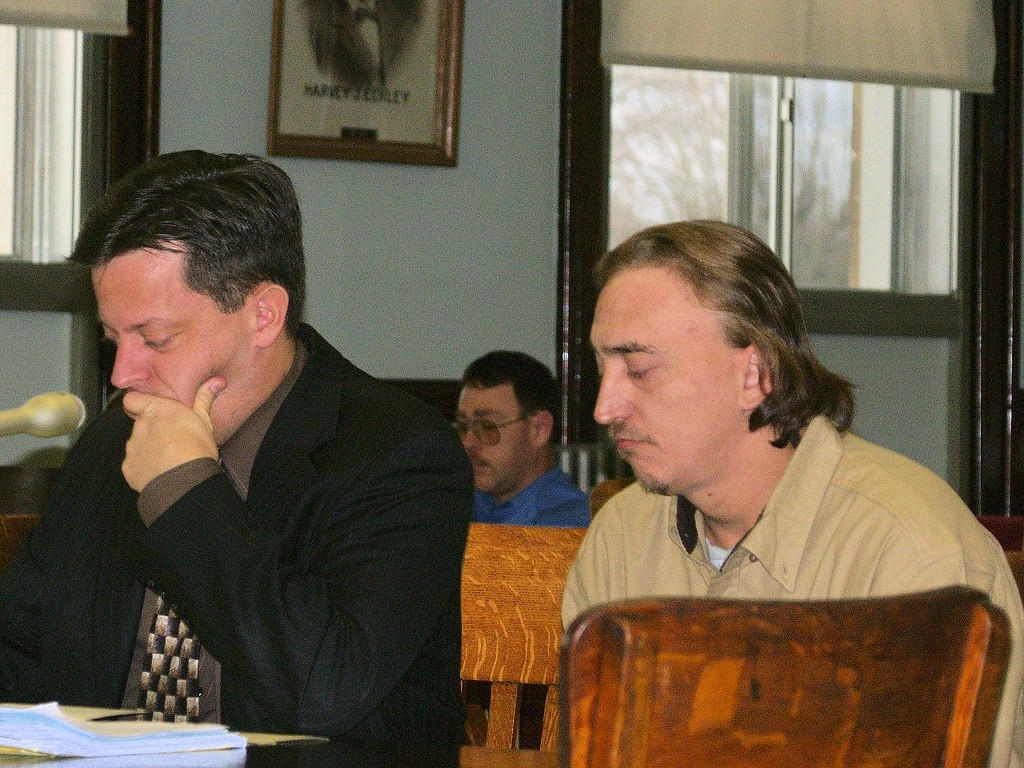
[561,218,1024,767]
[1,148,590,768]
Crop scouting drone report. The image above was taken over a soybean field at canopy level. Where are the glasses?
[450,411,530,445]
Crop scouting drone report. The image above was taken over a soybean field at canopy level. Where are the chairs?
[0,477,1024,768]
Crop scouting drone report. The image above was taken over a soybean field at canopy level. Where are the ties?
[140,593,203,724]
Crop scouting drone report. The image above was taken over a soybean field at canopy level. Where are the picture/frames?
[267,0,465,169]
